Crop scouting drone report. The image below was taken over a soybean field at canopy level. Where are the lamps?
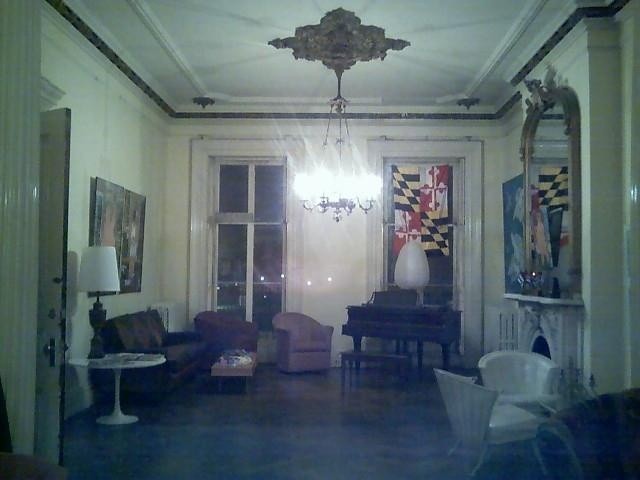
[267,5,410,223]
[395,241,430,289]
[75,245,121,358]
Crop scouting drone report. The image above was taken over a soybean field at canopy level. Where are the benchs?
[339,349,413,398]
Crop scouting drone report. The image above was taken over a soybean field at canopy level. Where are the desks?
[69,352,167,425]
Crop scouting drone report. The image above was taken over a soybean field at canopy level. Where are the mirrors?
[519,85,582,298]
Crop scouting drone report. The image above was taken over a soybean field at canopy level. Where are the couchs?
[103,309,208,403]
[271,312,334,373]
[193,311,259,373]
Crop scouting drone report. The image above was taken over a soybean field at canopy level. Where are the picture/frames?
[87,177,146,298]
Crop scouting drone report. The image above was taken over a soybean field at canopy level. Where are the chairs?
[479,351,561,457]
[433,367,549,477]
